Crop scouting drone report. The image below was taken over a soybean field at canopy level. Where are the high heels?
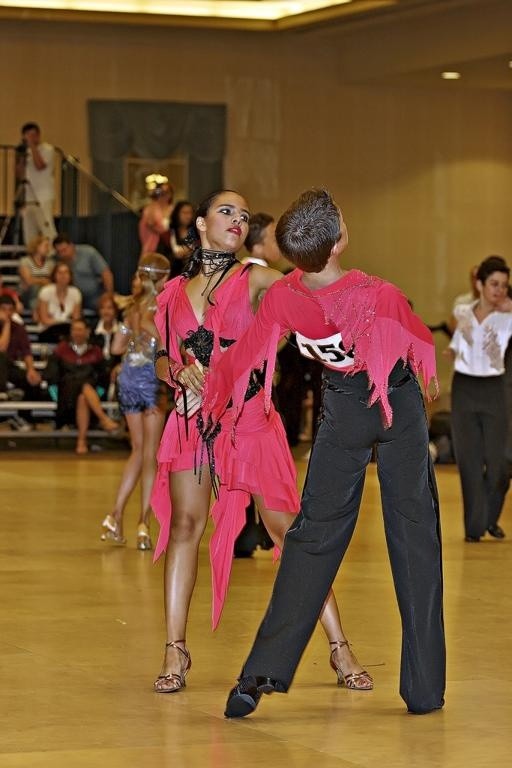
[136,523,152,550]
[328,639,375,691]
[154,639,193,693]
[101,514,128,545]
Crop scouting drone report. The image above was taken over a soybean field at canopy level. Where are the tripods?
[0,180,56,258]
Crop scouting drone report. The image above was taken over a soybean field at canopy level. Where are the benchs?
[0,235,132,449]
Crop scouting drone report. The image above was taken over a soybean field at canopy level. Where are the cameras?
[14,138,28,156]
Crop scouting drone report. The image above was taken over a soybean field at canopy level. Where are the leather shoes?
[488,525,505,539]
[224,675,276,717]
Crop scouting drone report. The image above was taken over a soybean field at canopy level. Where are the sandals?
[77,439,87,456]
[98,416,121,437]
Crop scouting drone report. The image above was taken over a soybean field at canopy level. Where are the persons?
[477,254,511,301]
[173,186,446,720]
[152,189,375,695]
[443,254,511,542]
[0,120,327,555]
[451,264,481,310]
